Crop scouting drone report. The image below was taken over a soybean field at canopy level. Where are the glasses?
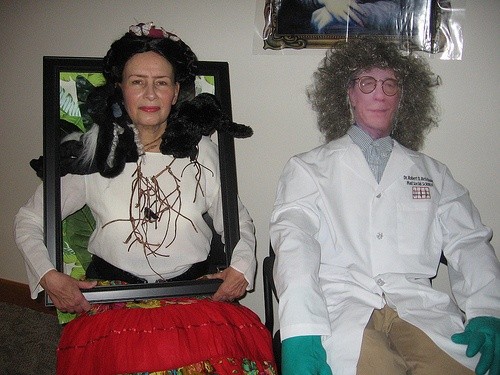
[352,75,402,97]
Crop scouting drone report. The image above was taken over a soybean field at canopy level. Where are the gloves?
[452,317,499,375]
[282,336,333,375]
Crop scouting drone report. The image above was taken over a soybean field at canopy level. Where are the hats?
[102,23,198,88]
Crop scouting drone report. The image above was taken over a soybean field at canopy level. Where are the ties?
[368,142,382,183]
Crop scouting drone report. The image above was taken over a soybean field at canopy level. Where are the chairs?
[262,227,451,375]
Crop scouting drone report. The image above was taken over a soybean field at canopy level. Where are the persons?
[268,34,500,375]
[13,23,277,375]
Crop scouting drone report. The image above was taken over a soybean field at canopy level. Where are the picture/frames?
[42,55,240,306]
[263,0,452,55]
